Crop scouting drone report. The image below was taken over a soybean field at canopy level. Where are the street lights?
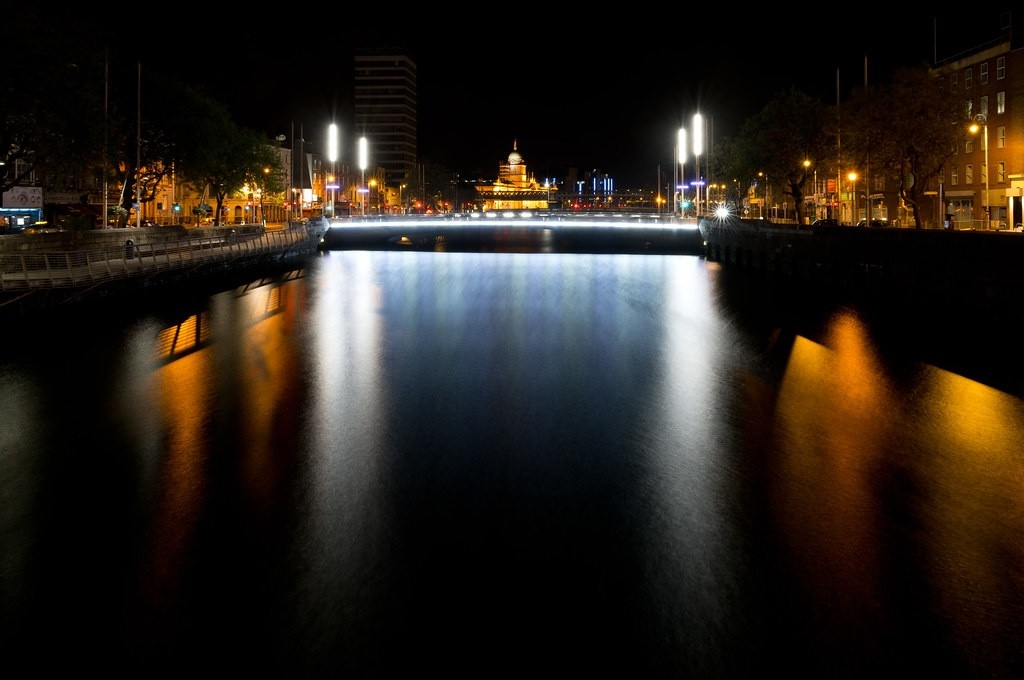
[968,114,990,231]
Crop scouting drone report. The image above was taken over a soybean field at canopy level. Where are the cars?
[810,218,844,227]
[132,220,160,228]
[857,218,895,228]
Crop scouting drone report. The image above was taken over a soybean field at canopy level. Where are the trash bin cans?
[125,239,136,260]
